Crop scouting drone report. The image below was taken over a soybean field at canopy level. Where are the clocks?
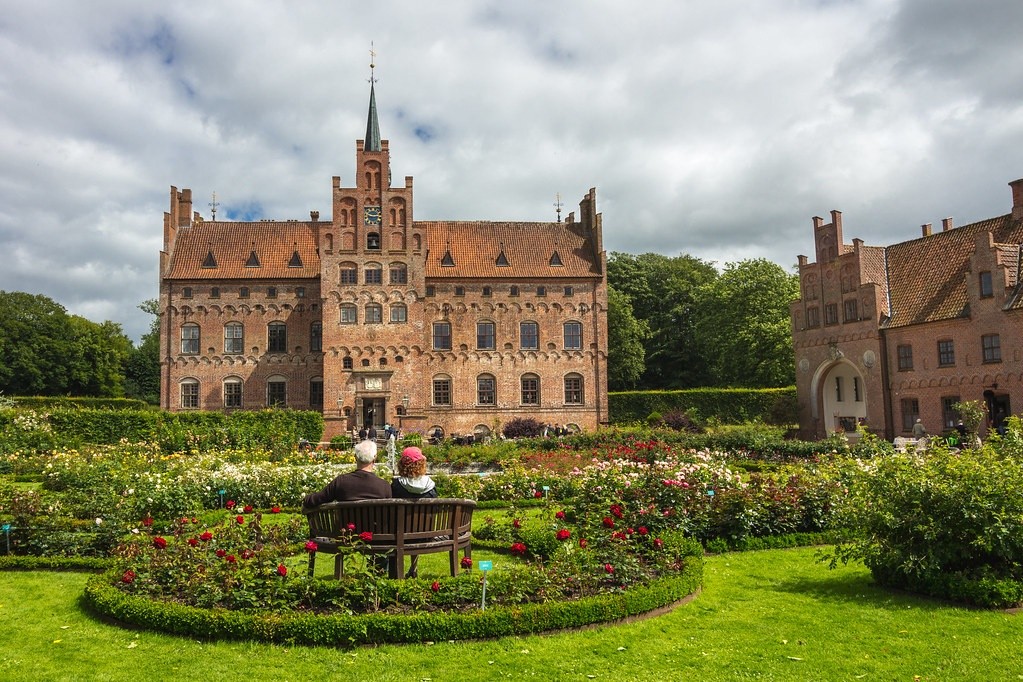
[364,209,382,226]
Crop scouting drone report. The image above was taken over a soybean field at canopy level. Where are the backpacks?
[560,428,562,433]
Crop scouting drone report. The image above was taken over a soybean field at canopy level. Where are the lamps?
[992,383,998,389]
[402,395,409,415]
[336,397,344,417]
[857,317,861,321]
[801,327,805,331]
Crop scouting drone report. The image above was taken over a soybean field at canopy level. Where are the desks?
[895,438,916,446]
[895,441,918,453]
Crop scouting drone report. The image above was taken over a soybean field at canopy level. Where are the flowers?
[825,343,841,362]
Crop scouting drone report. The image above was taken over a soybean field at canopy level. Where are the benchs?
[895,447,907,450]
[306,497,477,579]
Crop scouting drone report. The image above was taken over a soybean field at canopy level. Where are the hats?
[401,446,422,464]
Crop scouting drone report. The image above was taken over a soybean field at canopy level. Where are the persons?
[351,426,377,447]
[449,432,474,445]
[996,408,1007,434]
[955,420,965,436]
[391,448,436,579]
[304,441,393,576]
[913,419,926,441]
[539,423,568,439]
[434,428,441,440]
[385,423,395,438]
